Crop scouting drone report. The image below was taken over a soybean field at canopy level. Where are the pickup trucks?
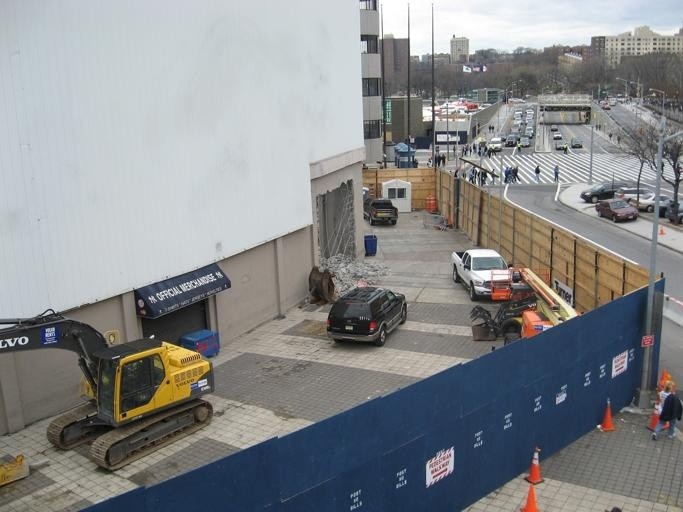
[362,197,398,227]
[445,249,512,302]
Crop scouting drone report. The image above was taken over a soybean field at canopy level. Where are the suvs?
[324,285,410,347]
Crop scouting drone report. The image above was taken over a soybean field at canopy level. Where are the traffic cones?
[519,484,543,512]
[523,447,545,485]
[658,225,664,234]
[645,368,676,434]
[598,402,616,432]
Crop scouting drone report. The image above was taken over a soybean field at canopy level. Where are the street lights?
[614,76,628,98]
[642,87,667,329]
[629,80,644,130]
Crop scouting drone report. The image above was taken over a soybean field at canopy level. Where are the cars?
[487,97,537,154]
[548,124,558,132]
[594,93,633,111]
[580,178,682,223]
[569,136,583,147]
[552,131,562,140]
[426,96,492,120]
[554,139,567,150]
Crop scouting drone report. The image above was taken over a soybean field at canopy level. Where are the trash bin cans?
[365,235,377,256]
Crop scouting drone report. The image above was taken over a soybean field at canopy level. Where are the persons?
[616,135,620,144]
[552,165,558,182]
[461,142,496,159]
[534,165,540,181]
[648,383,682,442]
[413,158,418,168]
[454,166,520,187]
[426,146,445,168]
[608,133,611,140]
[517,141,521,152]
[562,143,567,154]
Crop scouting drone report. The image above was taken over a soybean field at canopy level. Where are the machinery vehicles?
[0,305,220,489]
[467,263,582,351]
